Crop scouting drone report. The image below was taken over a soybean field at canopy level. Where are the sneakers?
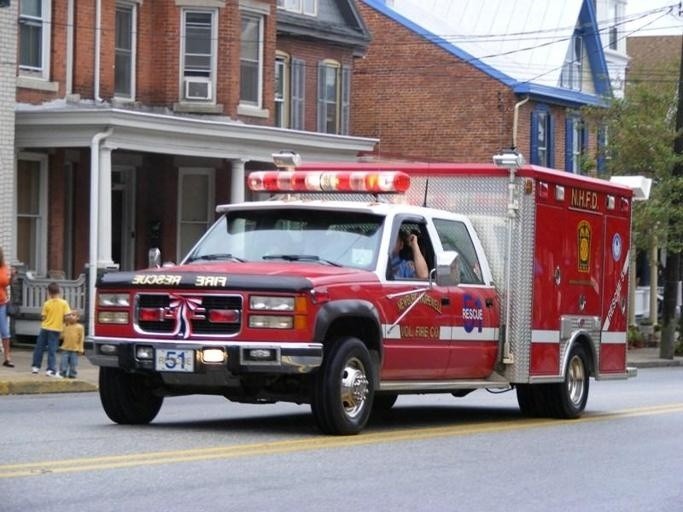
[32,365,76,379]
[3,359,14,367]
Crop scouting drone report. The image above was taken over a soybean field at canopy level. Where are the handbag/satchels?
[6,300,20,319]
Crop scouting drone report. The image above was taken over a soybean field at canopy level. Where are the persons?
[55,307,84,379]
[0,246,16,368]
[388,231,428,280]
[30,281,73,376]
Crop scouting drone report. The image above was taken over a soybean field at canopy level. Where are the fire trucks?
[78,145,653,439]
